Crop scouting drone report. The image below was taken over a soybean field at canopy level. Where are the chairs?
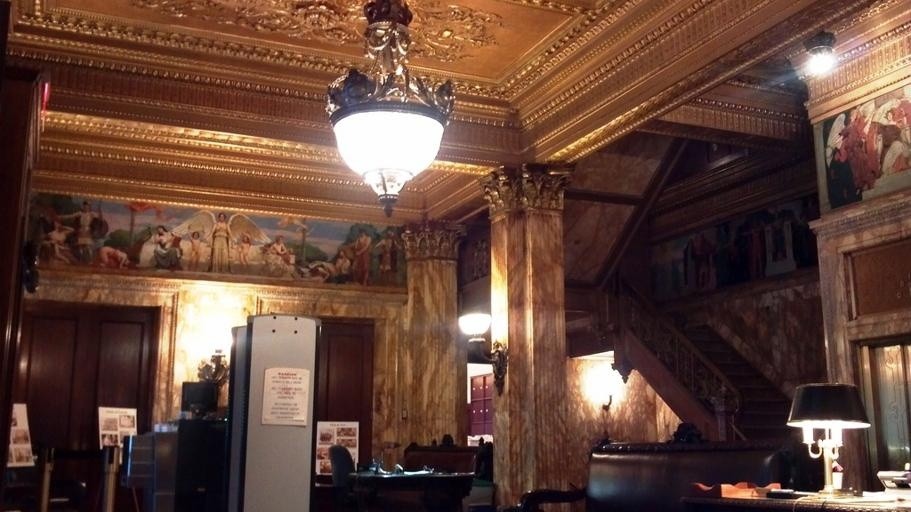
[327,445,377,512]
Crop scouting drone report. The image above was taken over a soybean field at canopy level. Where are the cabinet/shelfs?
[470,374,495,437]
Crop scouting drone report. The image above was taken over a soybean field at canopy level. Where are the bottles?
[832,459,845,490]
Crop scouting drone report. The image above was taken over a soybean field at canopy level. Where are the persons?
[826,90,911,208]
[21,200,409,286]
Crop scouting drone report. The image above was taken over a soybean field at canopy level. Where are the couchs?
[516,443,792,511]
[383,446,479,512]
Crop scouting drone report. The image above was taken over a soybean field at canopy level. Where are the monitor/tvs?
[182,381,218,420]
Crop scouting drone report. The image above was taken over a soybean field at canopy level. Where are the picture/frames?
[26,186,408,294]
[633,185,821,307]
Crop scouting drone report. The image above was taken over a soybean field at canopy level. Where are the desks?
[175,420,227,512]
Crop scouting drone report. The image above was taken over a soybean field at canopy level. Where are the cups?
[154,421,178,432]
[183,411,193,420]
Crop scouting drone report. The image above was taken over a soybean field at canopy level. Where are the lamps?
[786,383,871,498]
[457,313,509,397]
[802,30,839,78]
[323,0,456,218]
